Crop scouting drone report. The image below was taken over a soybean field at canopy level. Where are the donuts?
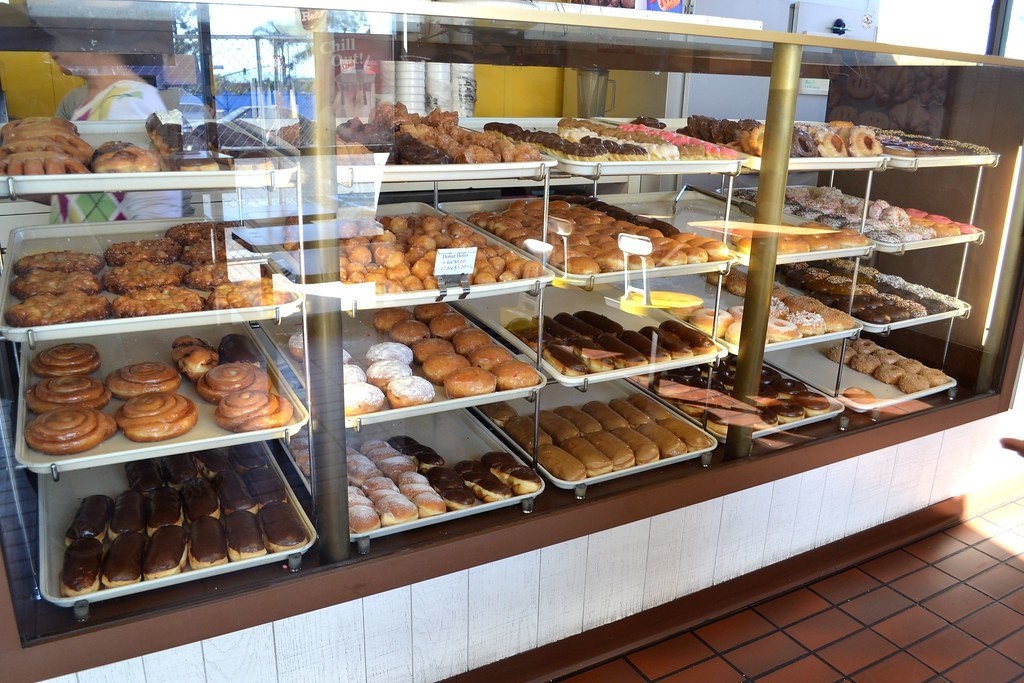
[628,115,992,436]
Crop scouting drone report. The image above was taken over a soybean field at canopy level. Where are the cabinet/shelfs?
[0,1,1024,683]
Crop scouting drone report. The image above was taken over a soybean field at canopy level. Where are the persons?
[49,51,182,225]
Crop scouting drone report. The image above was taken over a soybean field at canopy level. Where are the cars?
[178,102,225,121]
[193,106,314,147]
[183,113,193,140]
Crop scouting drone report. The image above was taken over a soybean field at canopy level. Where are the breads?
[0,99,738,593]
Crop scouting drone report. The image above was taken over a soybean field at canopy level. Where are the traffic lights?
[243,68,246,75]
